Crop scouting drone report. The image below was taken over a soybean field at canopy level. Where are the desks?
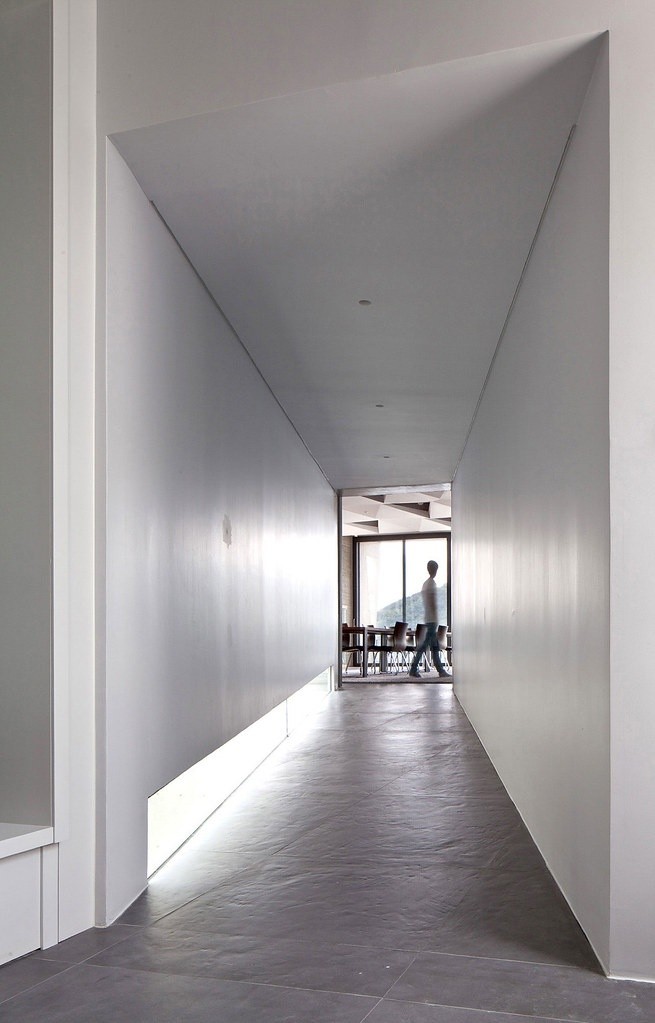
[343,627,451,678]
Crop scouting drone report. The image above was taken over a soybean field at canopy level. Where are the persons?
[409,560,452,677]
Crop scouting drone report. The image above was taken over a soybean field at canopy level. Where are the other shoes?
[409,672,422,678]
[439,670,452,677]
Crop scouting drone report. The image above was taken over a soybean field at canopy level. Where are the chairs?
[368,621,409,675]
[393,623,431,673]
[342,623,364,677]
[431,625,450,671]
[362,624,384,675]
